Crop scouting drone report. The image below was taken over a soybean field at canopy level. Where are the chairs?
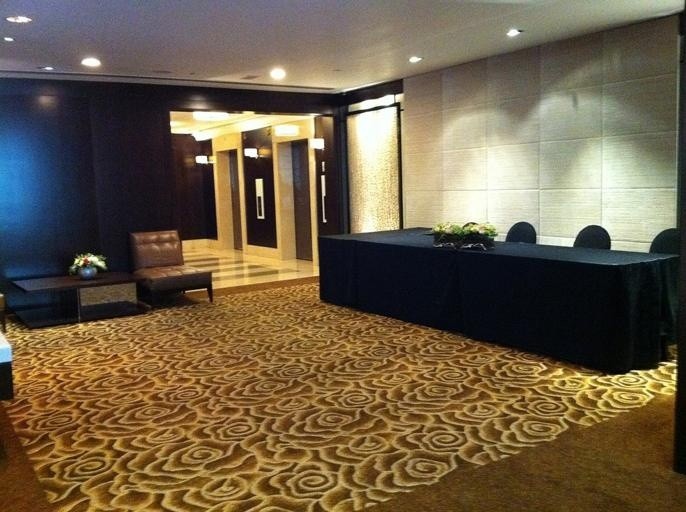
[128,228,214,307]
[461,222,682,257]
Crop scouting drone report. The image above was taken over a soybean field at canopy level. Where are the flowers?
[433,221,496,237]
[68,252,107,271]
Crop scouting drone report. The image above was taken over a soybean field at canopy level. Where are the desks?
[316,227,682,375]
[11,270,145,329]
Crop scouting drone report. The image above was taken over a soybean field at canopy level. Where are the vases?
[434,232,492,251]
[78,265,98,279]
